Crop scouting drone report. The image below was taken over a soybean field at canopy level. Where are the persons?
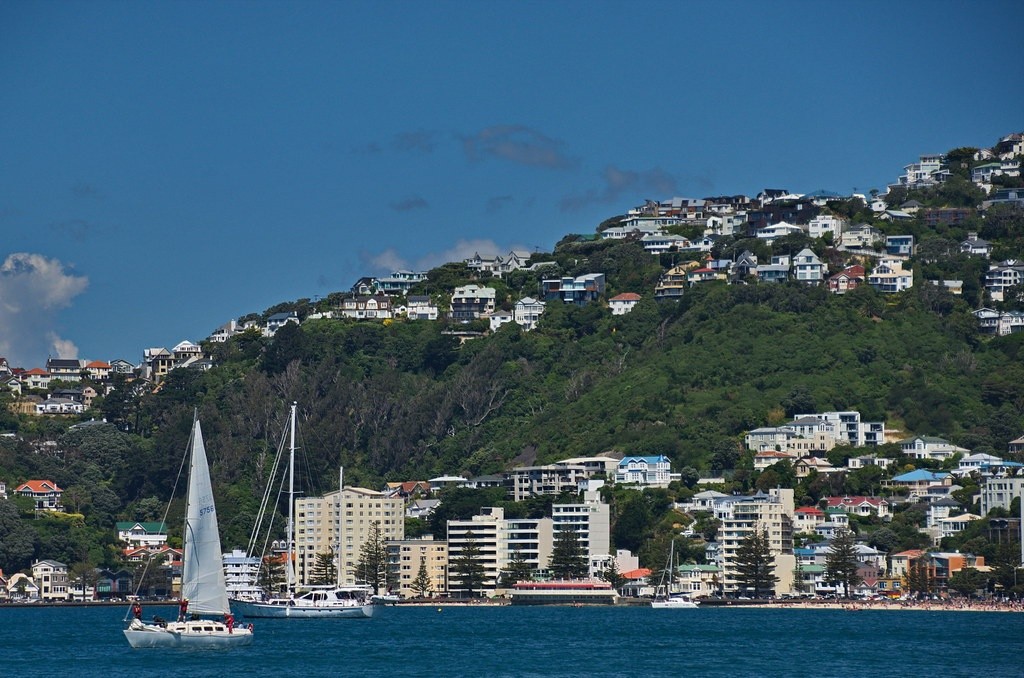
[248,624,252,632]
[133,603,141,620]
[177,597,189,622]
[224,613,234,635]
[781,598,1024,612]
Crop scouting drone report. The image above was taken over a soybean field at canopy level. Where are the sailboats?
[228,399,374,619]
[649,539,698,608]
[370,564,400,606]
[124,405,257,652]
